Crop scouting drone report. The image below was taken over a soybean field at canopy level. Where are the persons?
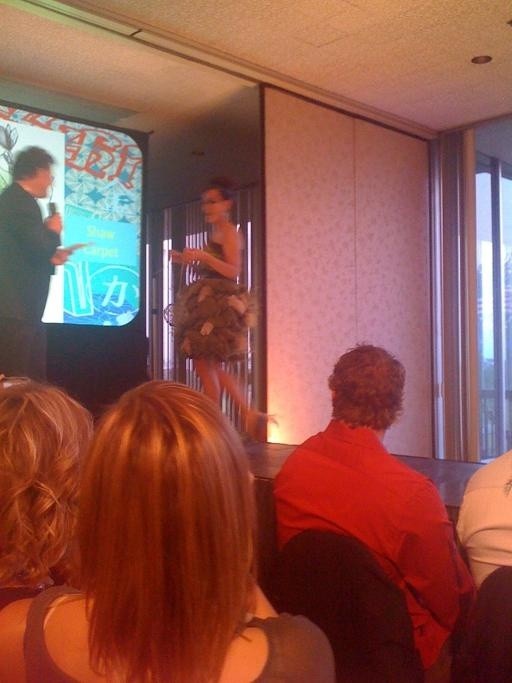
[163,175,262,444]
[273,342,475,683]
[0,141,98,382]
[0,371,89,608]
[454,441,511,587]
[0,377,341,682]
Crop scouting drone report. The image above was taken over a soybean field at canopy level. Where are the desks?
[247,438,491,525]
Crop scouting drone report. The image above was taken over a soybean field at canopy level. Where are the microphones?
[49,203,55,215]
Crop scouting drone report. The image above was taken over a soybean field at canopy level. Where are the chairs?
[271,530,511,680]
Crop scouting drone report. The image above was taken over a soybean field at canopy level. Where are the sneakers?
[239,410,268,446]
